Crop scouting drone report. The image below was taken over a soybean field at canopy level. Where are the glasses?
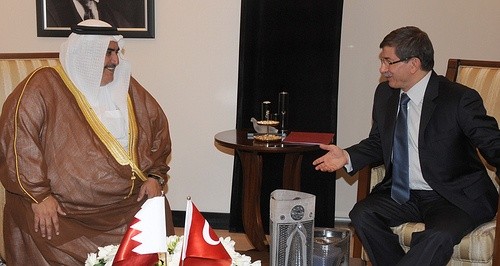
[379,55,423,68]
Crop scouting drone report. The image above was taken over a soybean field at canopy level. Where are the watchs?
[148,175,164,185]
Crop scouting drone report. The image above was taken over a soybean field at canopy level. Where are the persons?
[47,0,136,28]
[313,25,500,266]
[0,20,171,266]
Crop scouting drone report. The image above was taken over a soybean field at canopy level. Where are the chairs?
[353,59,500,266]
[0,53,61,263]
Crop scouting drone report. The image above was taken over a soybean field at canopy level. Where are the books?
[283,132,335,145]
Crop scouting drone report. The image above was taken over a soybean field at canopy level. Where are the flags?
[179,200,232,266]
[111,195,168,266]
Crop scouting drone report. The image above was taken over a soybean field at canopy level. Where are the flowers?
[85,236,262,266]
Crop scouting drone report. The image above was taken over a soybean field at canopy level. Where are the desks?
[214,128,334,251]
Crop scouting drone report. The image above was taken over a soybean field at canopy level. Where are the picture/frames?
[36,0,156,38]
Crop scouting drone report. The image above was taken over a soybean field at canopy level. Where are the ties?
[78,0,94,20]
[390,93,411,205]
